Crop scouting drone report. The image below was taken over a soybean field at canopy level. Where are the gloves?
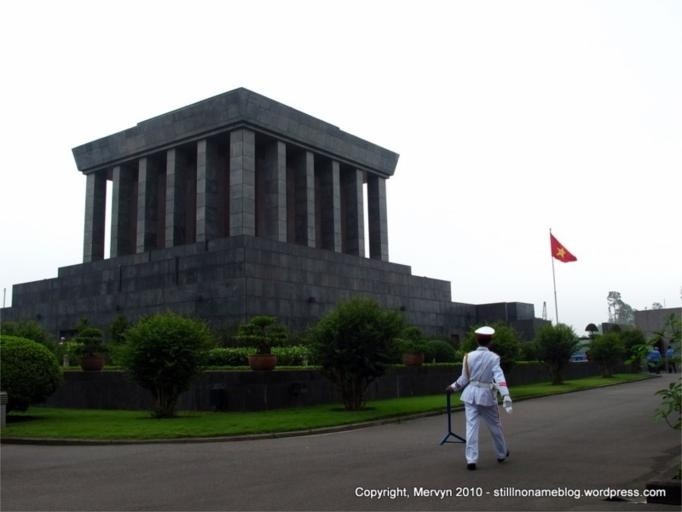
[445,385,455,393]
[502,396,513,415]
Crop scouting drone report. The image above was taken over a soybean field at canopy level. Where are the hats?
[475,326,495,336]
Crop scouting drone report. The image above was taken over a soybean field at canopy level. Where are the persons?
[646,345,662,374]
[444,324,517,472]
[665,345,678,374]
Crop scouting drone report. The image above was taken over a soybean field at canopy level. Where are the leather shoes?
[497,448,509,464]
[466,462,477,471]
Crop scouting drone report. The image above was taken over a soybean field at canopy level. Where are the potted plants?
[68,328,109,370]
[228,311,288,370]
[399,325,428,365]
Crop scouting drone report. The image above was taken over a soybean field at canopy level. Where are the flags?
[549,232,577,264]
